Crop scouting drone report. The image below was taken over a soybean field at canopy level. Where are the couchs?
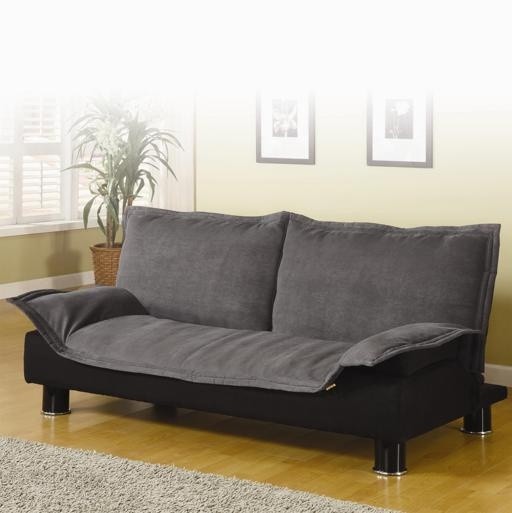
[4,207,508,476]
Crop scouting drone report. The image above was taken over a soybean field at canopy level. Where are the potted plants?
[62,99,184,285]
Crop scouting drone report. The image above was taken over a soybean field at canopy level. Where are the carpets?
[0,437,399,512]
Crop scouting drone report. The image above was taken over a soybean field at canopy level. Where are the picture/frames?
[366,86,433,167]
[254,90,315,165]
